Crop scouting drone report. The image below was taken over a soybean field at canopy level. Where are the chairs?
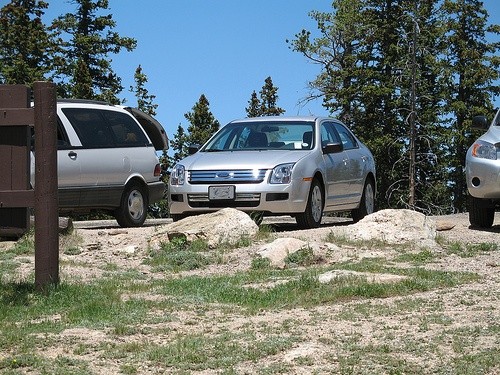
[242,130,324,153]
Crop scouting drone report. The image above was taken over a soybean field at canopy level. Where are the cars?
[463,108,499,231]
[168,114,381,230]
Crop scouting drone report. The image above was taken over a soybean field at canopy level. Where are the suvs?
[16,97,169,227]
